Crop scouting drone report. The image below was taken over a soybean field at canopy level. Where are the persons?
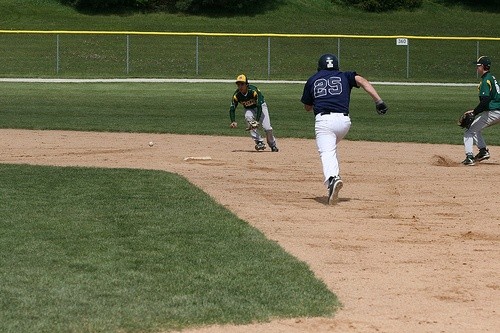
[300,54,389,205]
[229,74,279,152]
[459,55,500,166]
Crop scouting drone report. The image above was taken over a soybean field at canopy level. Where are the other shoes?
[271,146,280,152]
[327,175,344,205]
[255,142,267,151]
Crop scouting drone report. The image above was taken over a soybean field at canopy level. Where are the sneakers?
[475,149,491,162]
[462,155,480,166]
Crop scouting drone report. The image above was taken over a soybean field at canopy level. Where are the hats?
[474,56,492,67]
[235,74,248,84]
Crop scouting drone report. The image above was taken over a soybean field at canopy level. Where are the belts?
[320,111,350,116]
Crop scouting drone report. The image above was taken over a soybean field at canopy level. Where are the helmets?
[318,54,339,71]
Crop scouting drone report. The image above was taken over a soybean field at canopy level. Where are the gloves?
[375,99,388,115]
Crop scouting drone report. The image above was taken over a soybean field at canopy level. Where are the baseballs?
[148,141,154,147]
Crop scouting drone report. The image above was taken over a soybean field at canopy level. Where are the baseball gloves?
[245,121,260,132]
[459,109,474,130]
